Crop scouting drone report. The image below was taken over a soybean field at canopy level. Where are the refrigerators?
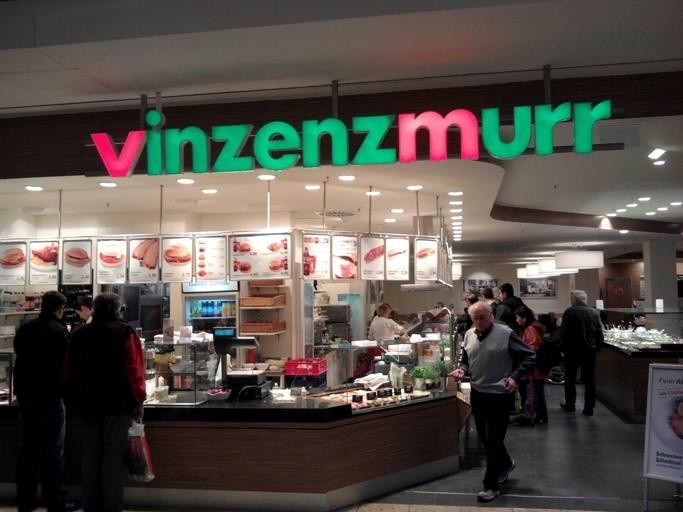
[180,273,248,368]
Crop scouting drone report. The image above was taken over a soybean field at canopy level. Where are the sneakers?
[560,399,575,411]
[498,460,515,483]
[582,407,594,416]
[477,489,500,500]
[510,415,548,424]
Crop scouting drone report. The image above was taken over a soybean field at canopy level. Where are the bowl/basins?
[206,388,231,402]
[242,362,268,371]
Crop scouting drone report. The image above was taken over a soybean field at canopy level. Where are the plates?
[29,260,56,271]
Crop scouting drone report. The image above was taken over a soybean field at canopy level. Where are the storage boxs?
[284,359,327,389]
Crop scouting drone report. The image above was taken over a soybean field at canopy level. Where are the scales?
[214,328,273,402]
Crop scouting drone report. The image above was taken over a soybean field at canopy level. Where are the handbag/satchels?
[536,336,560,373]
[126,420,156,483]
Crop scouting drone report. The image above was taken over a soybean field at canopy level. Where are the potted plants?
[446,360,457,390]
[430,362,444,388]
[409,366,428,396]
[440,341,451,356]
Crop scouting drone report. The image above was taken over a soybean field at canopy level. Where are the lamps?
[516,250,604,280]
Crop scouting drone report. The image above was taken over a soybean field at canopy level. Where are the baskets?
[241,321,284,332]
[240,294,285,306]
[285,358,327,376]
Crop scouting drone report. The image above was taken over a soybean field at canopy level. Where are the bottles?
[191,300,236,317]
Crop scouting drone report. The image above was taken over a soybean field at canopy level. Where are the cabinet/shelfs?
[143,341,218,406]
[0,350,18,407]
[0,311,40,348]
[238,284,292,389]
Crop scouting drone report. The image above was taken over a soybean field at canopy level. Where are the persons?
[372,304,408,343]
[454,283,496,336]
[495,283,535,415]
[14,291,71,511]
[435,301,443,310]
[368,302,392,341]
[72,294,96,326]
[446,300,537,502]
[65,292,146,512]
[555,288,604,415]
[513,306,552,429]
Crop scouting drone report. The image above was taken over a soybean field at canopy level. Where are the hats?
[571,289,587,304]
[94,294,121,313]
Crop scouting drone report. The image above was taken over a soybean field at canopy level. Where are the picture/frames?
[517,280,558,299]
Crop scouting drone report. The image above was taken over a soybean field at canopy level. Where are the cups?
[246,347,257,363]
[179,325,193,344]
[161,317,174,342]
